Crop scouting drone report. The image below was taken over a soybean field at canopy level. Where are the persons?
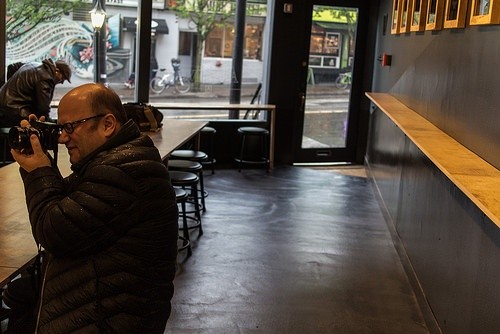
[11,84,179,334]
[0,58,71,127]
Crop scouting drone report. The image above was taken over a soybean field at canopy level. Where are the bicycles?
[335,72,352,90]
[150,59,191,94]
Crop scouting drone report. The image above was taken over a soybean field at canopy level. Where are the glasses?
[57,114,108,135]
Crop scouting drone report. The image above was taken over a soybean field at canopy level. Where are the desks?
[0,121,210,334]
[49,100,276,171]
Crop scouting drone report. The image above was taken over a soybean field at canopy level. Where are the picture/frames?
[400,0,413,33]
[469,0,500,25]
[391,0,401,34]
[410,0,427,32]
[425,0,444,30]
[443,0,468,29]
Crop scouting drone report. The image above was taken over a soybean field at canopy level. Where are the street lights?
[89,0,107,83]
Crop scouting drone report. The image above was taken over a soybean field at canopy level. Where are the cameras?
[8,119,59,155]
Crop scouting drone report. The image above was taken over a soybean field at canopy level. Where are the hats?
[54,59,73,85]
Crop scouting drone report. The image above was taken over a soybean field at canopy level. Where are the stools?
[167,171,203,236]
[168,160,200,218]
[187,126,216,175]
[170,150,209,213]
[173,188,192,258]
[234,127,270,173]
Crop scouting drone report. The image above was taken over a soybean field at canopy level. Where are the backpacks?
[121,100,165,132]
